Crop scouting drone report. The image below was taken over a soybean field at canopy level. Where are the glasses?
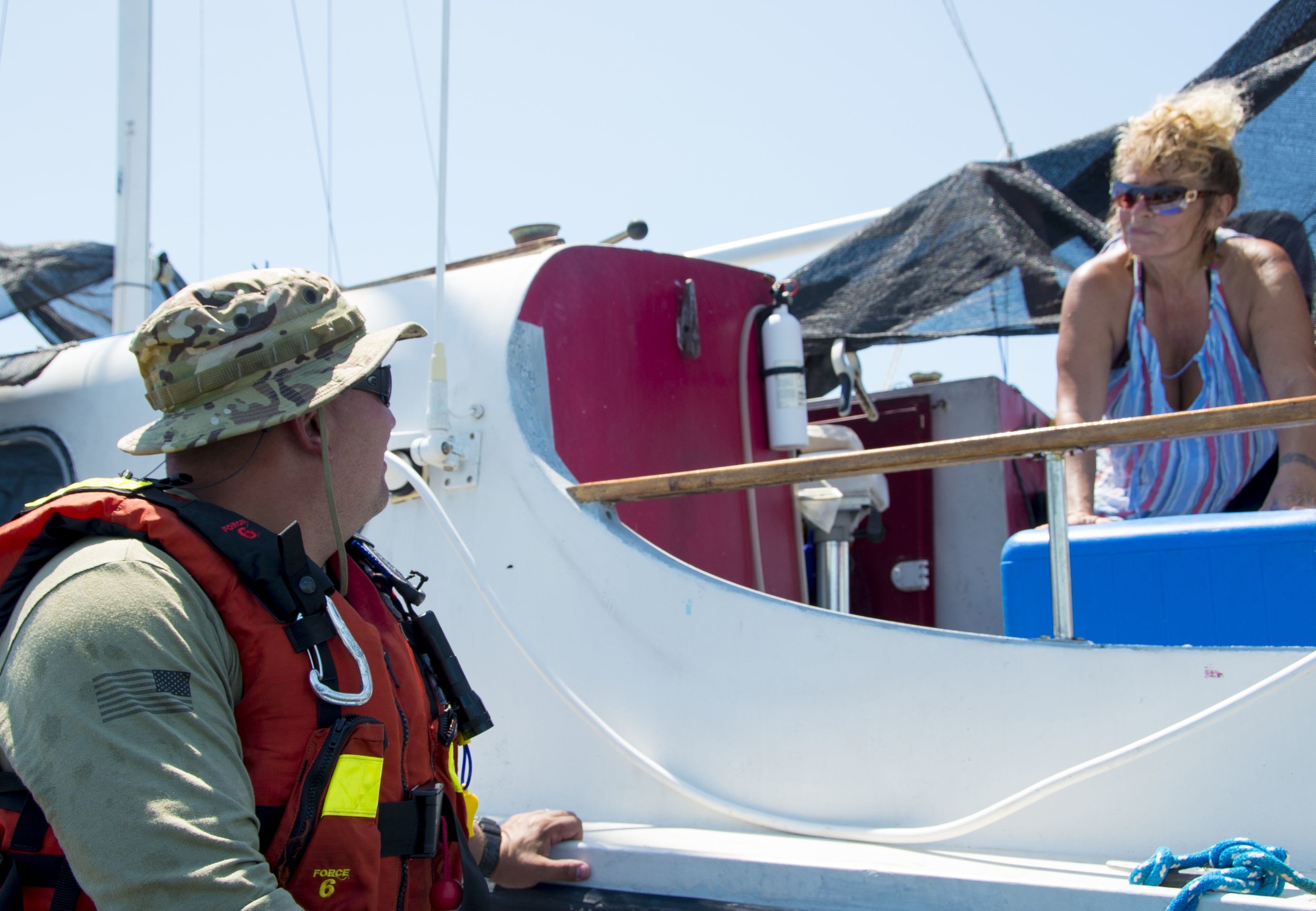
[352,365,392,408]
[1109,179,1223,214]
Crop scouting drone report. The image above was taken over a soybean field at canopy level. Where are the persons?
[0,268,590,911]
[1056,74,1316,526]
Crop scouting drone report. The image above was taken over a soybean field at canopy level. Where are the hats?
[118,268,429,456]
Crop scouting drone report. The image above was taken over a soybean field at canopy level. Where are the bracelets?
[478,817,502,879]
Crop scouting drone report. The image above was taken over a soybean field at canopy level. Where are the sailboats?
[0,2,1316,911]
